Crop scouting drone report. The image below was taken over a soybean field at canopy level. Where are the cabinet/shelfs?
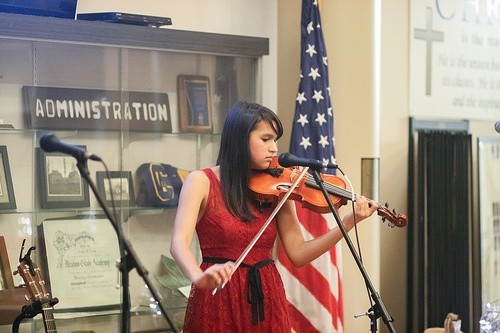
[0,13,269,333]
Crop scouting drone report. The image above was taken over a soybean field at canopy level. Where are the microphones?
[40,132,102,161]
[278,152,338,169]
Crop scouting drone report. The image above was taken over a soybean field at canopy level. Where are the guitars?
[12,260,57,333]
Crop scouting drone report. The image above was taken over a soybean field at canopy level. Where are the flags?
[274,0,345,333]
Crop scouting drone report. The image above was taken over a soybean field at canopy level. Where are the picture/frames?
[0,145,17,210]
[176,73,214,134]
[96,170,135,207]
[34,144,90,209]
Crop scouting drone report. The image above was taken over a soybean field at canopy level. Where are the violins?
[247,156,408,229]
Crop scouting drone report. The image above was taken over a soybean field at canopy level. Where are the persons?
[170,102,378,333]
